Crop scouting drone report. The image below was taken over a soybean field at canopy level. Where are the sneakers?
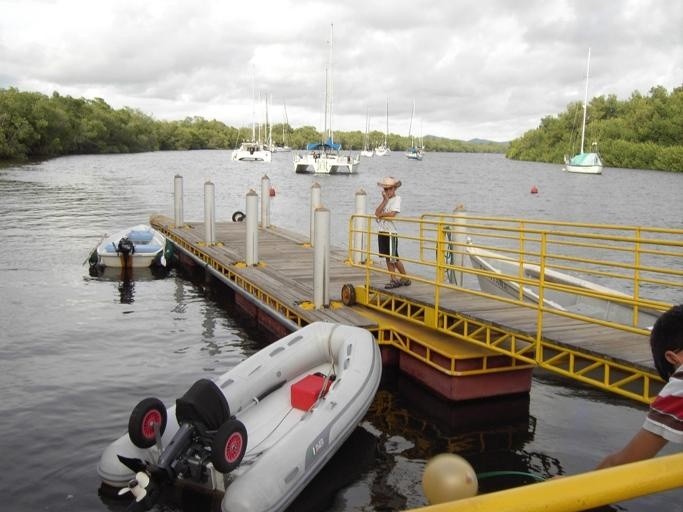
[399,278,411,286]
[384,279,399,288]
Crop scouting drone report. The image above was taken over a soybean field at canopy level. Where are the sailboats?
[404,97,425,159]
[374,95,390,156]
[293,24,359,174]
[232,64,290,162]
[559,47,604,175]
[360,107,373,157]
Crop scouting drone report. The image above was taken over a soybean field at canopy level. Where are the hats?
[378,176,401,189]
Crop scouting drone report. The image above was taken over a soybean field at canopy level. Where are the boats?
[94,320,382,512]
[86,226,166,276]
[466,236,658,331]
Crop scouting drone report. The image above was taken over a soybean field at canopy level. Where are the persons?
[541,304,682,480]
[374,176,412,289]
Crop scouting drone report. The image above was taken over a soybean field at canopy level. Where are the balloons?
[421,452,478,506]
